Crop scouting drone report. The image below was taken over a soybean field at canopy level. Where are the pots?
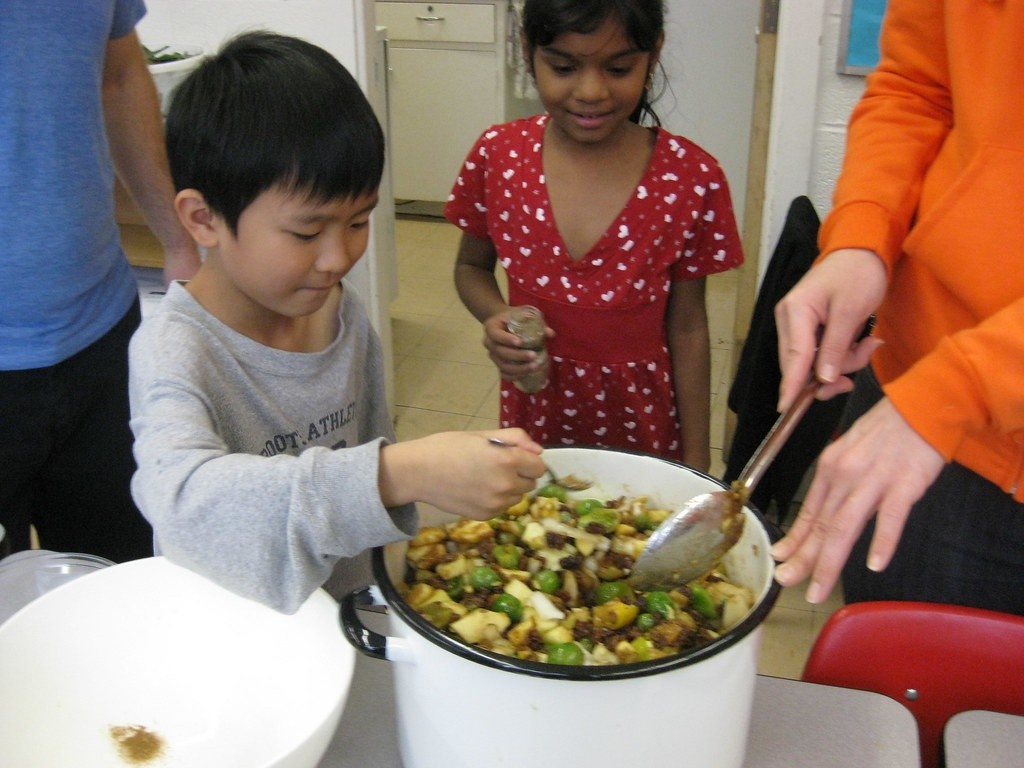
[340,443,785,768]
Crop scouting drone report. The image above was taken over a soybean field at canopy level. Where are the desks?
[319,639,919,767]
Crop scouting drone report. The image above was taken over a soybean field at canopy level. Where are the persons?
[445,0,746,476]
[768,0,1024,618]
[126,32,544,615]
[0,0,202,565]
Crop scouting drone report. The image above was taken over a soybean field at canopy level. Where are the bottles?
[507,306,550,393]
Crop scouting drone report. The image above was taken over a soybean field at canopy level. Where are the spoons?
[628,370,823,590]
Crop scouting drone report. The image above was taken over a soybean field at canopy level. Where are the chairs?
[801,601,1024,768]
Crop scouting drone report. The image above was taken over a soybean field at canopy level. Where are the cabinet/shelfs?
[376,1,537,218]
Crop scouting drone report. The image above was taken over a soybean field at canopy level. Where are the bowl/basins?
[0,555,355,768]
